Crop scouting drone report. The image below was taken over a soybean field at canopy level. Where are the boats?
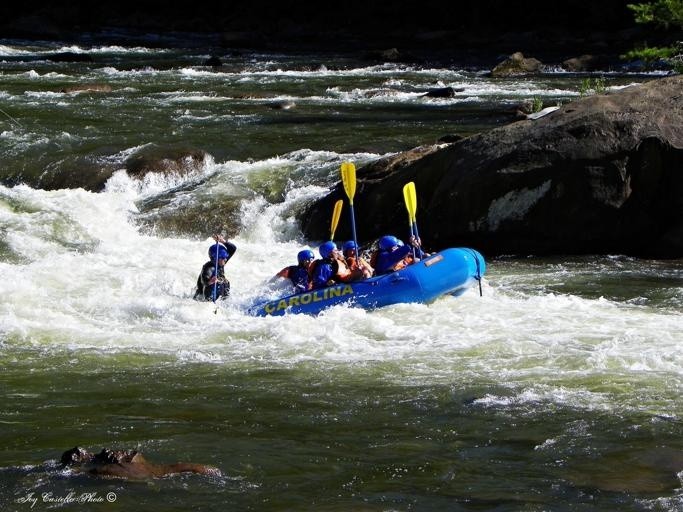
[239,245,486,316]
[241,248,486,331]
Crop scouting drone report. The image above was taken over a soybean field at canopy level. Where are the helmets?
[298,250,314,261]
[343,241,358,252]
[397,239,405,247]
[209,244,229,259]
[379,236,397,250]
[319,241,336,259]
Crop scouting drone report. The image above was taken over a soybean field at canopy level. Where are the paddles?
[402,181,423,261]
[340,161,358,265]
[330,199,343,243]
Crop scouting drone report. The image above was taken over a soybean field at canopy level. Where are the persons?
[371,235,417,275]
[394,237,430,260]
[193,234,237,303]
[288,250,315,295]
[314,241,345,290]
[342,241,360,259]
